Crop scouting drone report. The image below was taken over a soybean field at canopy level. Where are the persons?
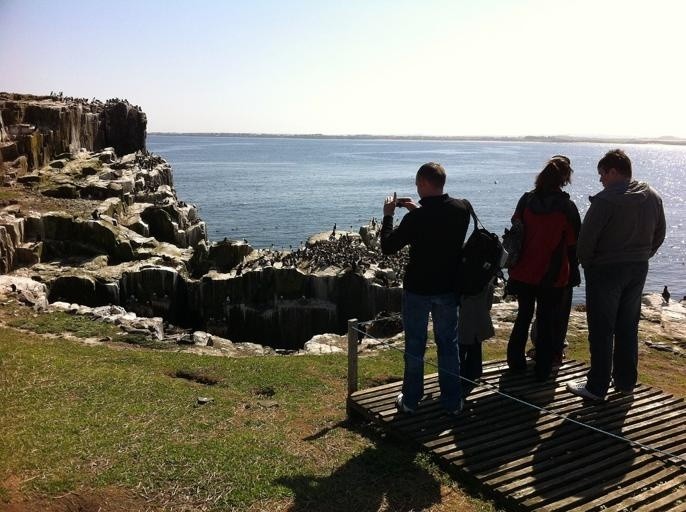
[47,90,143,112]
[223,216,410,288]
[380,162,470,416]
[566,150,667,401]
[460,279,495,382]
[506,155,581,381]
[108,149,203,230]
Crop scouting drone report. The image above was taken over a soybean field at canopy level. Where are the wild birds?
[661,284,671,305]
[91,208,99,219]
[232,223,408,287]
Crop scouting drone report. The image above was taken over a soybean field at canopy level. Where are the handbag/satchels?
[457,227,503,297]
[502,220,526,255]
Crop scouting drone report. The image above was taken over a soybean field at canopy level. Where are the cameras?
[395,197,411,208]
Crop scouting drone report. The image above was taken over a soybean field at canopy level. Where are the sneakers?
[612,378,634,395]
[398,393,415,413]
[448,398,464,415]
[566,381,601,401]
